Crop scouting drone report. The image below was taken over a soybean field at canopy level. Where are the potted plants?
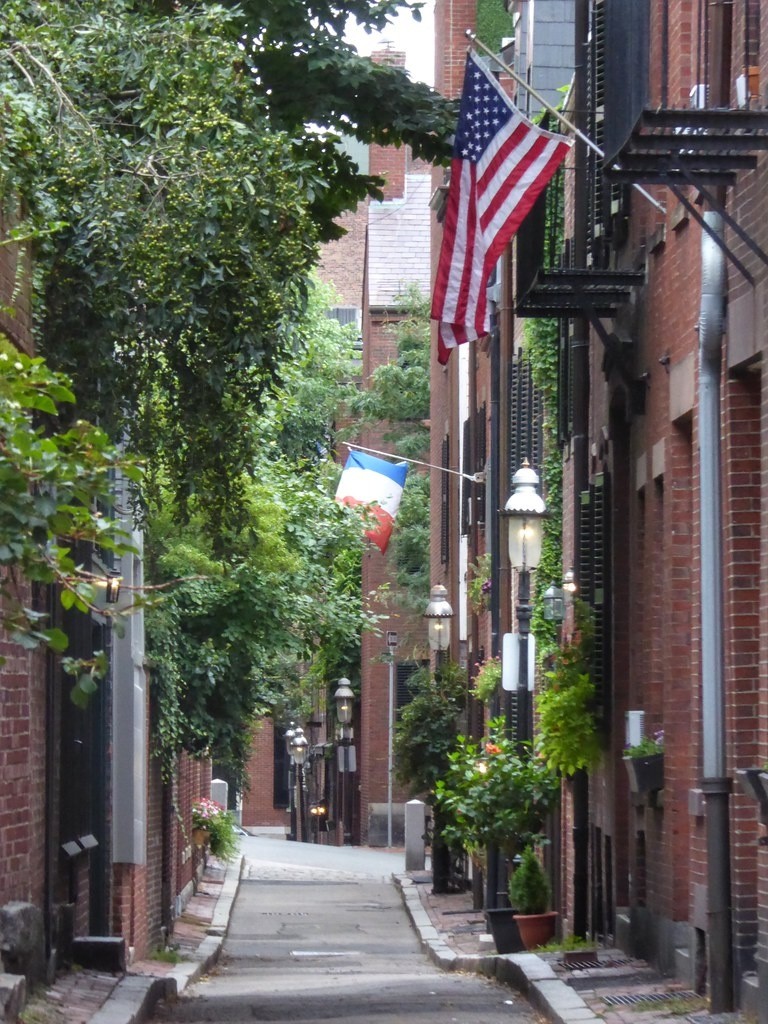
[509,849,557,948]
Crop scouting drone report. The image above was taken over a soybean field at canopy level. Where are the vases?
[485,910,524,956]
[624,754,664,794]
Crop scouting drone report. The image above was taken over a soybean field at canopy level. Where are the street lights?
[486,456,548,937]
[282,721,298,841]
[421,580,456,895]
[291,725,315,842]
[333,673,358,844]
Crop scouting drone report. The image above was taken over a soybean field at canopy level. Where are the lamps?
[542,583,568,623]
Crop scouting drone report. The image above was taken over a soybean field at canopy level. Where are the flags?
[429,46,576,366]
[334,450,408,556]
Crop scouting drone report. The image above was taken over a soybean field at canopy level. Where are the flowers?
[620,731,664,757]
[192,798,225,826]
[431,718,560,908]
